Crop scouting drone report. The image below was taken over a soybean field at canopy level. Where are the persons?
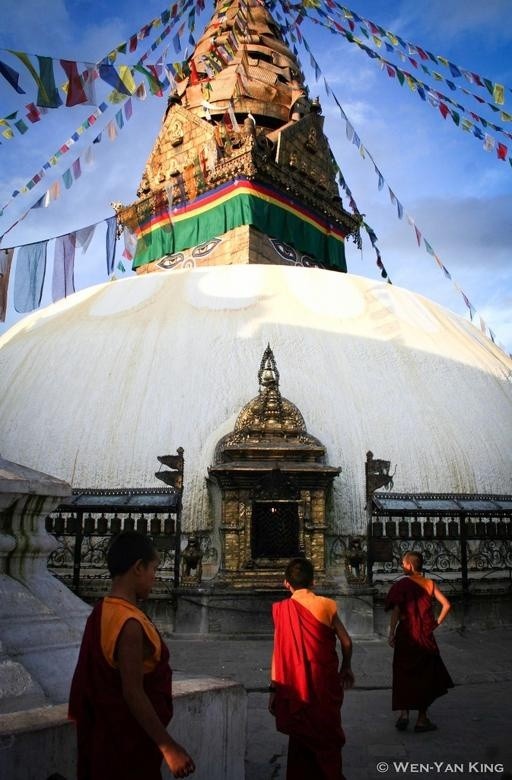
[267,558,352,780]
[381,550,455,734]
[67,531,197,779]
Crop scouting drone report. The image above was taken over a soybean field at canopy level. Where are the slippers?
[395,717,410,730]
[414,719,439,732]
